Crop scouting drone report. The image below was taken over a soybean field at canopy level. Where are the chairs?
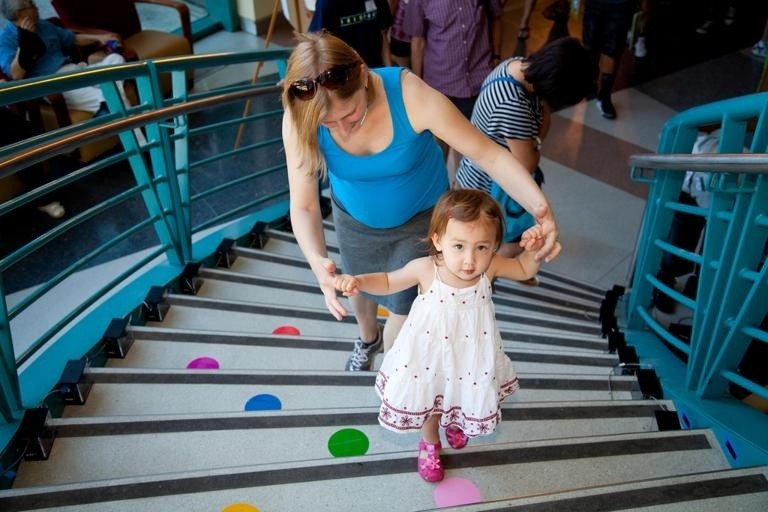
[0,1,196,206]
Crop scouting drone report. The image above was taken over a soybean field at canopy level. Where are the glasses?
[287,57,361,101]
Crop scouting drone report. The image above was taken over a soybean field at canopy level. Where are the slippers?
[518,27,530,40]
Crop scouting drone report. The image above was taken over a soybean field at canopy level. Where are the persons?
[453,35,599,285]
[1,106,66,219]
[0,0,147,150]
[332,188,545,482]
[282,32,563,370]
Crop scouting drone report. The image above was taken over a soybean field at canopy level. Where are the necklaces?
[360,110,368,126]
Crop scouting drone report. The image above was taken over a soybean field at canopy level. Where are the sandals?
[445,423,468,451]
[418,435,445,482]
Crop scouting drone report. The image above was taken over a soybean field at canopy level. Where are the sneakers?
[595,93,618,120]
[345,323,384,371]
[652,270,680,315]
[634,36,648,58]
